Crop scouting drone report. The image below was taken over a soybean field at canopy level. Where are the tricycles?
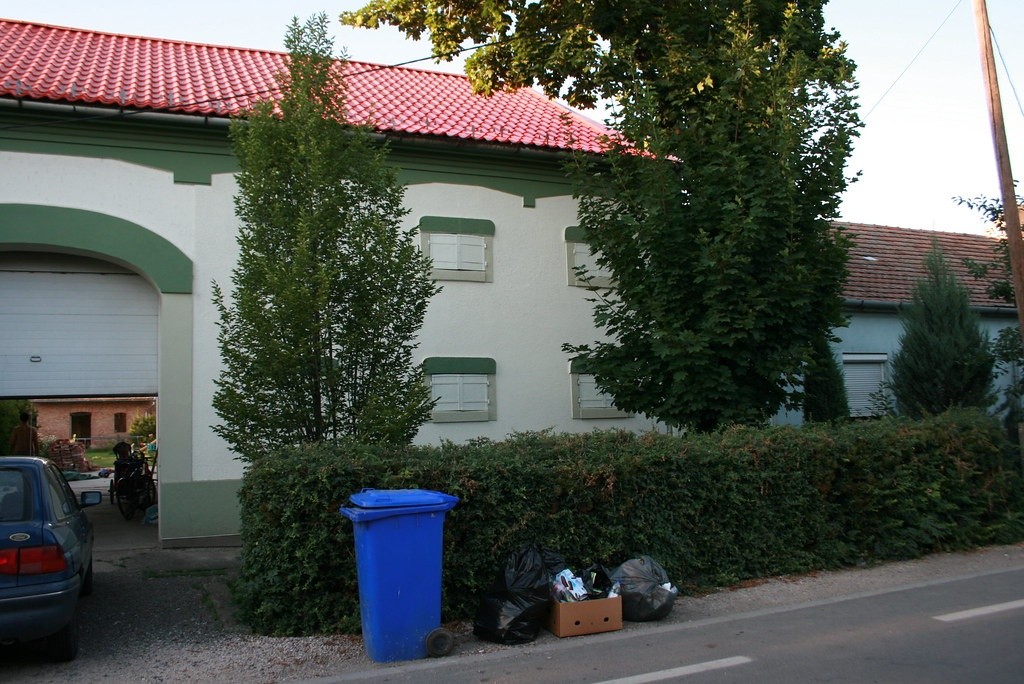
[110,458,155,520]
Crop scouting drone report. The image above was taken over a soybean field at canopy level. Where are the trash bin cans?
[339,488,460,663]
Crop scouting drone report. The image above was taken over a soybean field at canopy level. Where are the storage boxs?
[549,595,623,638]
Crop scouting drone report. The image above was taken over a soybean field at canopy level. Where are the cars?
[0,455,103,662]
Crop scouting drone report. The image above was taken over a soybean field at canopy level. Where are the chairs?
[0,492,23,520]
